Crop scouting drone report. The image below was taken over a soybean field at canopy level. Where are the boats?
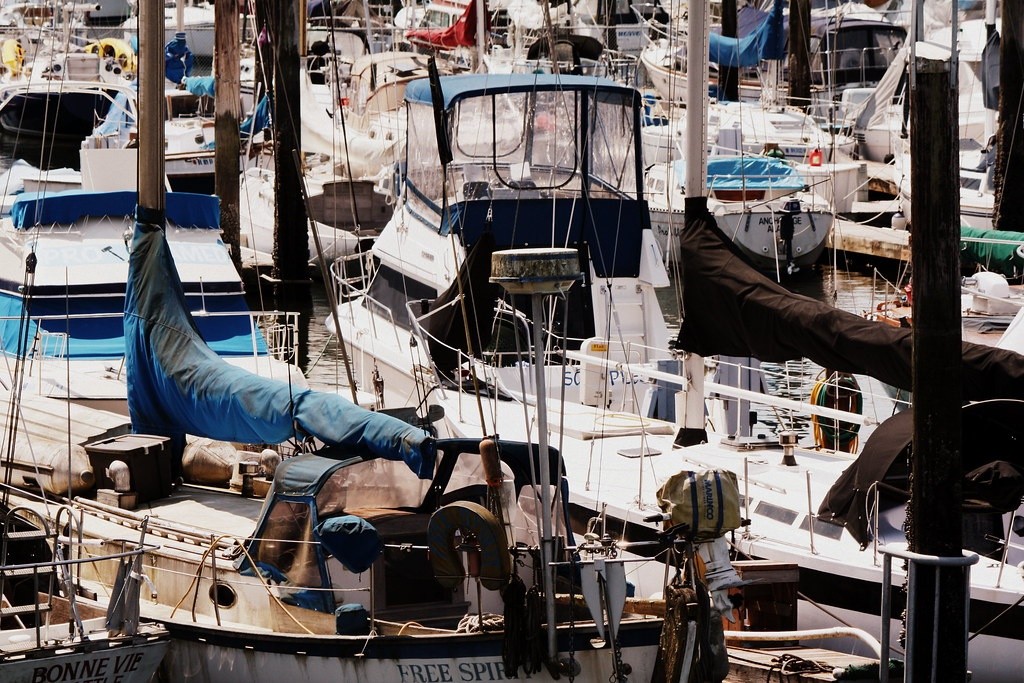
[0,0,1024,683]
